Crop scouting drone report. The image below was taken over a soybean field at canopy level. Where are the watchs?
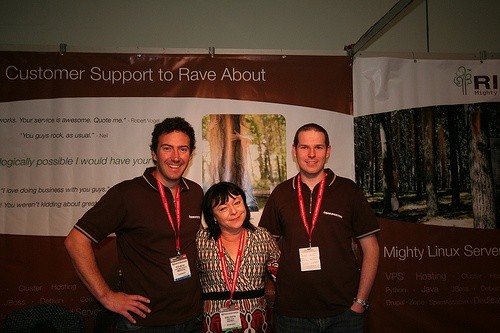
[353,297,370,309]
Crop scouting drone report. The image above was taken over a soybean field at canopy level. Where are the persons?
[193,181,282,333]
[258,122,382,333]
[64,117,205,333]
